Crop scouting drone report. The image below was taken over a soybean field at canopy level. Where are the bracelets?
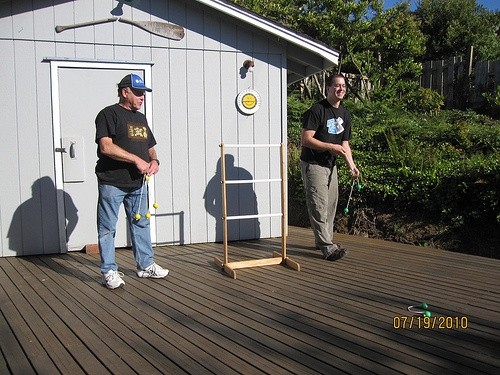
[150,159,160,166]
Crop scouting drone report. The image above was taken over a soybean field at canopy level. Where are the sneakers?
[102,269,125,290]
[136,262,170,278]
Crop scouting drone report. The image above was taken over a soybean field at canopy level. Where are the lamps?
[243,59,254,69]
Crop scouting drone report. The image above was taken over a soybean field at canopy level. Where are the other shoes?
[328,248,349,261]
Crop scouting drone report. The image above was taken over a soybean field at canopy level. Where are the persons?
[300,74,360,262]
[94,73,170,289]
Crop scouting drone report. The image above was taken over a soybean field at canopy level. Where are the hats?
[119,73,152,92]
